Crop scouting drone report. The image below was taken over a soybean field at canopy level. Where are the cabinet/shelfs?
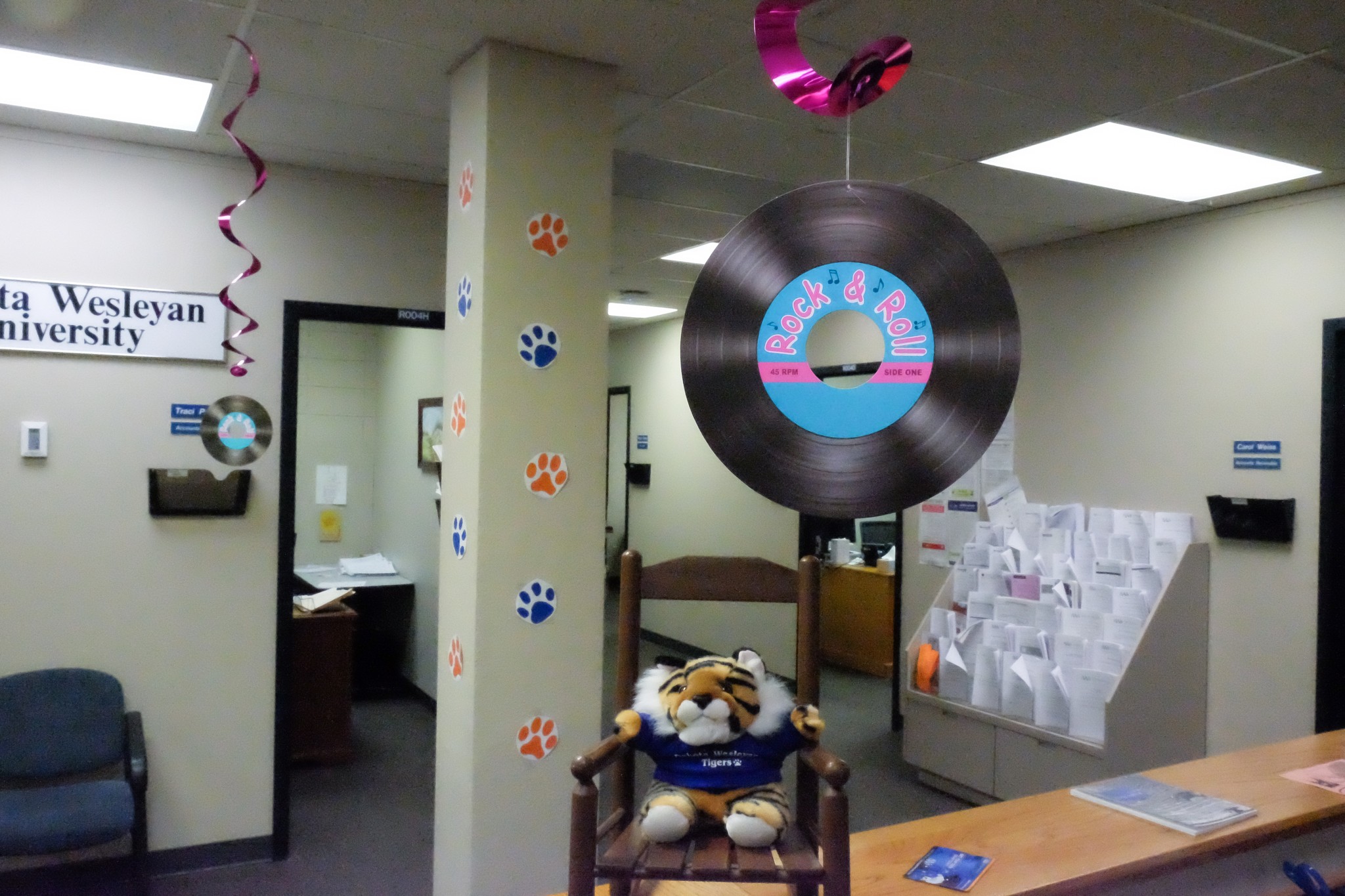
[902,537,1211,805]
[818,562,895,676]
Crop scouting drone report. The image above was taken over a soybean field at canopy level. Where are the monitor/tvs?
[855,513,897,555]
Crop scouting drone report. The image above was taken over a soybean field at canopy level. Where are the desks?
[294,563,415,696]
[294,604,359,767]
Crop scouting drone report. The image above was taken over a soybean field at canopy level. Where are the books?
[1069,776,1257,836]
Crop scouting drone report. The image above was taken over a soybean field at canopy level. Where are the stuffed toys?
[614,649,825,848]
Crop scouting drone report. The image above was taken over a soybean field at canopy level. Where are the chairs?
[568,549,850,896]
[0,668,152,896]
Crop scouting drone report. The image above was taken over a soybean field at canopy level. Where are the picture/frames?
[417,398,443,471]
